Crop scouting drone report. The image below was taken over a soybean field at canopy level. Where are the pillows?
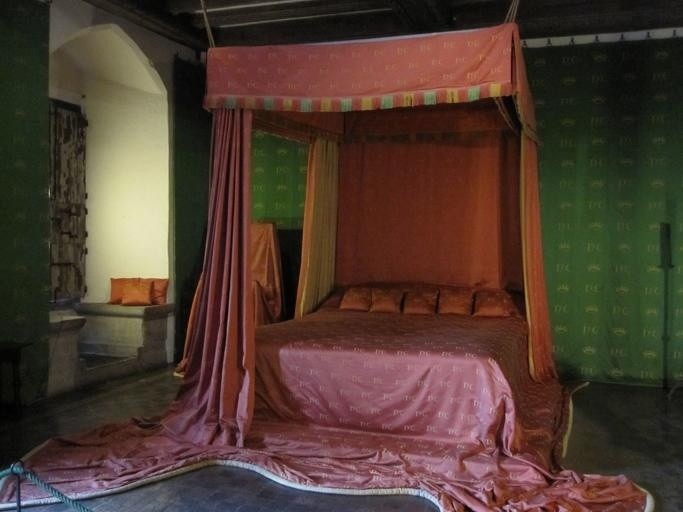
[341,285,511,317]
[109,277,169,304]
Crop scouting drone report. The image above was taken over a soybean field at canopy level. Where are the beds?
[0,0,656,511]
